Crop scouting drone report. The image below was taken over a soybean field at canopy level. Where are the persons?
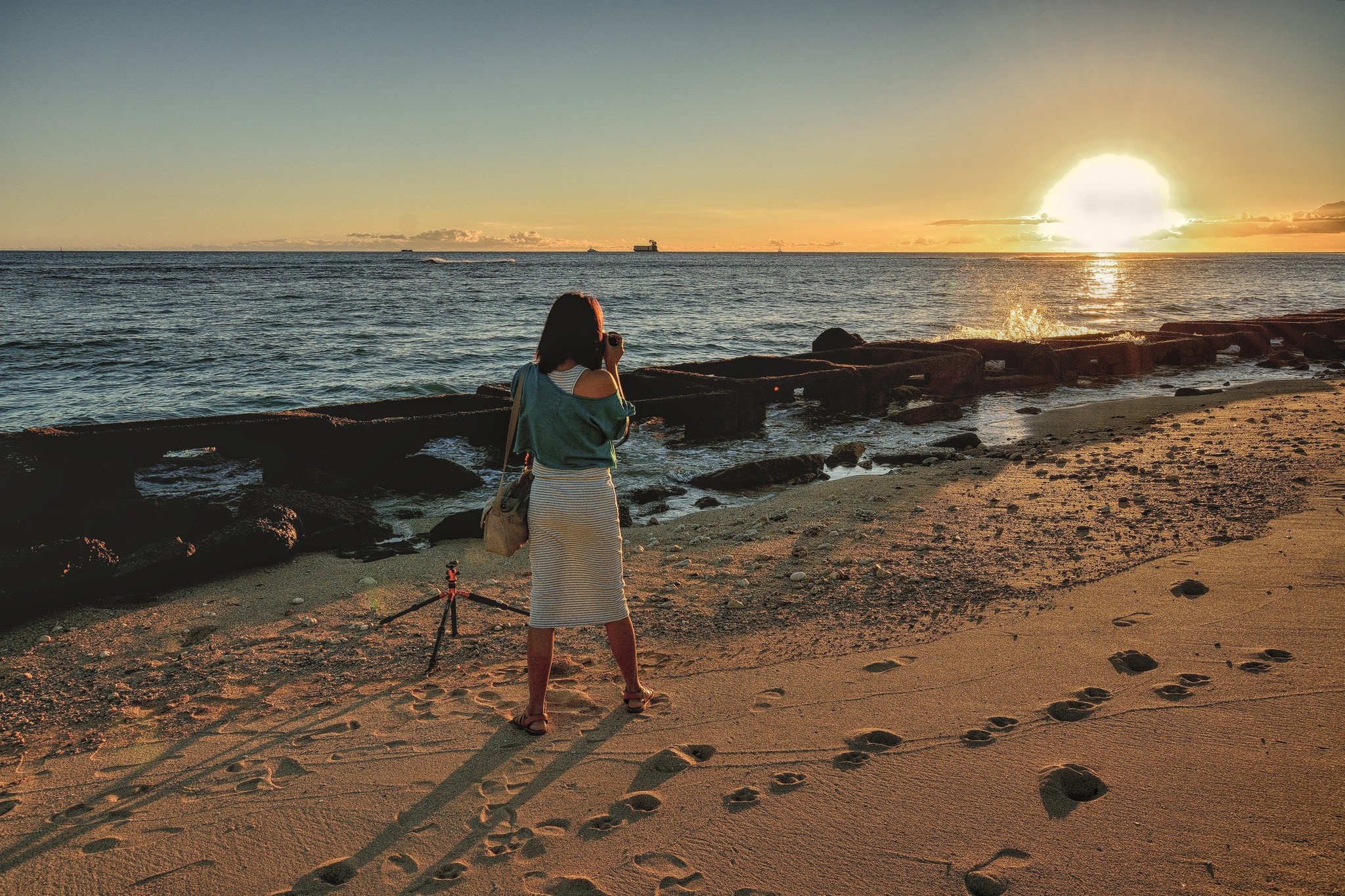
[510,293,655,736]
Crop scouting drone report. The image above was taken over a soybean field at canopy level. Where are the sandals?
[512,700,549,736]
[623,681,654,713]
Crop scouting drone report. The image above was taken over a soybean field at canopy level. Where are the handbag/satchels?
[481,469,535,557]
